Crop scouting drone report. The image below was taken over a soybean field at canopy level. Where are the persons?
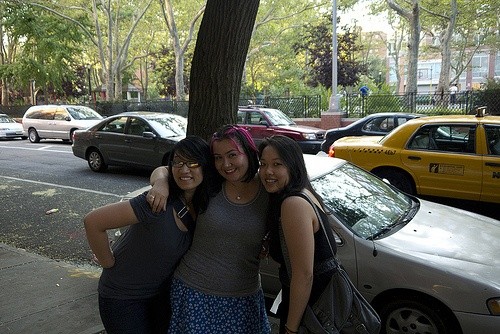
[430,82,490,108]
[259,134,382,334]
[83,136,210,334]
[146,125,275,334]
[158,89,189,112]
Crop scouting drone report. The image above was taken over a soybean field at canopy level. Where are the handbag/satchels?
[296,267,381,334]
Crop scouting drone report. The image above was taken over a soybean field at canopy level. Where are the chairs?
[238,116,242,122]
[108,124,117,130]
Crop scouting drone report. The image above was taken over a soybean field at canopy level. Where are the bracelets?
[285,324,298,334]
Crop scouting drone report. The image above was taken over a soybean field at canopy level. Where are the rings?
[149,195,155,199]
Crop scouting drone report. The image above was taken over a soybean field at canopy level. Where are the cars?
[320,111,469,154]
[112,152,500,334]
[328,105,500,205]
[0,114,29,140]
[71,111,188,179]
[234,104,326,156]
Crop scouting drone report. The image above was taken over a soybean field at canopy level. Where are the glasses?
[212,124,234,137]
[171,159,201,168]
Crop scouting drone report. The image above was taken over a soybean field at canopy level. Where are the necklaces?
[229,180,247,200]
[183,201,193,211]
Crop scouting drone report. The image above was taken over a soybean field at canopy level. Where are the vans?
[22,103,123,145]
